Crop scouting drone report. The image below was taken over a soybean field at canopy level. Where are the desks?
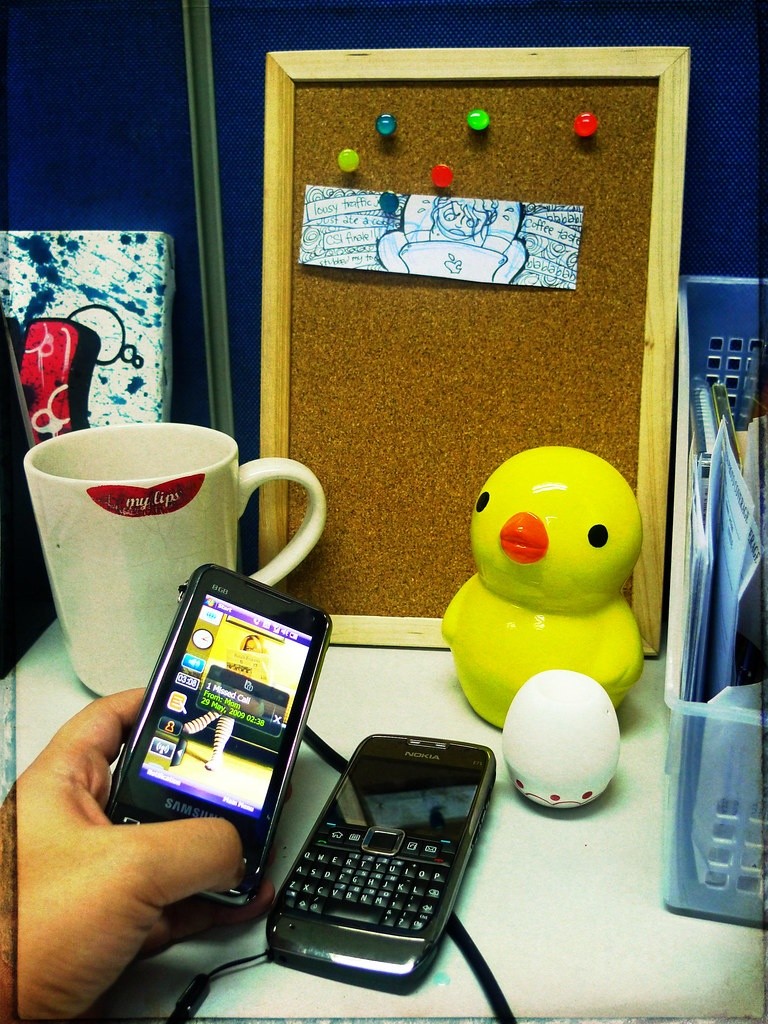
[2,619,767,1024]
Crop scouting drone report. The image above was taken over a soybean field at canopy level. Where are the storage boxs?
[667,275,767,927]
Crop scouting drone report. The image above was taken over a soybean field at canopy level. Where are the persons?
[183,634,271,771]
[0,686,293,1024]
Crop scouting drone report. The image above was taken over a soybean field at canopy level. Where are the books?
[667,382,764,904]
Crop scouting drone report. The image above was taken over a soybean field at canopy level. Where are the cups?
[23,422,326,698]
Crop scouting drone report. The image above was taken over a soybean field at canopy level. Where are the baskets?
[659,274,768,923]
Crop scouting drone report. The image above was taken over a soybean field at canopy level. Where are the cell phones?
[100,563,334,910]
[267,732,496,996]
